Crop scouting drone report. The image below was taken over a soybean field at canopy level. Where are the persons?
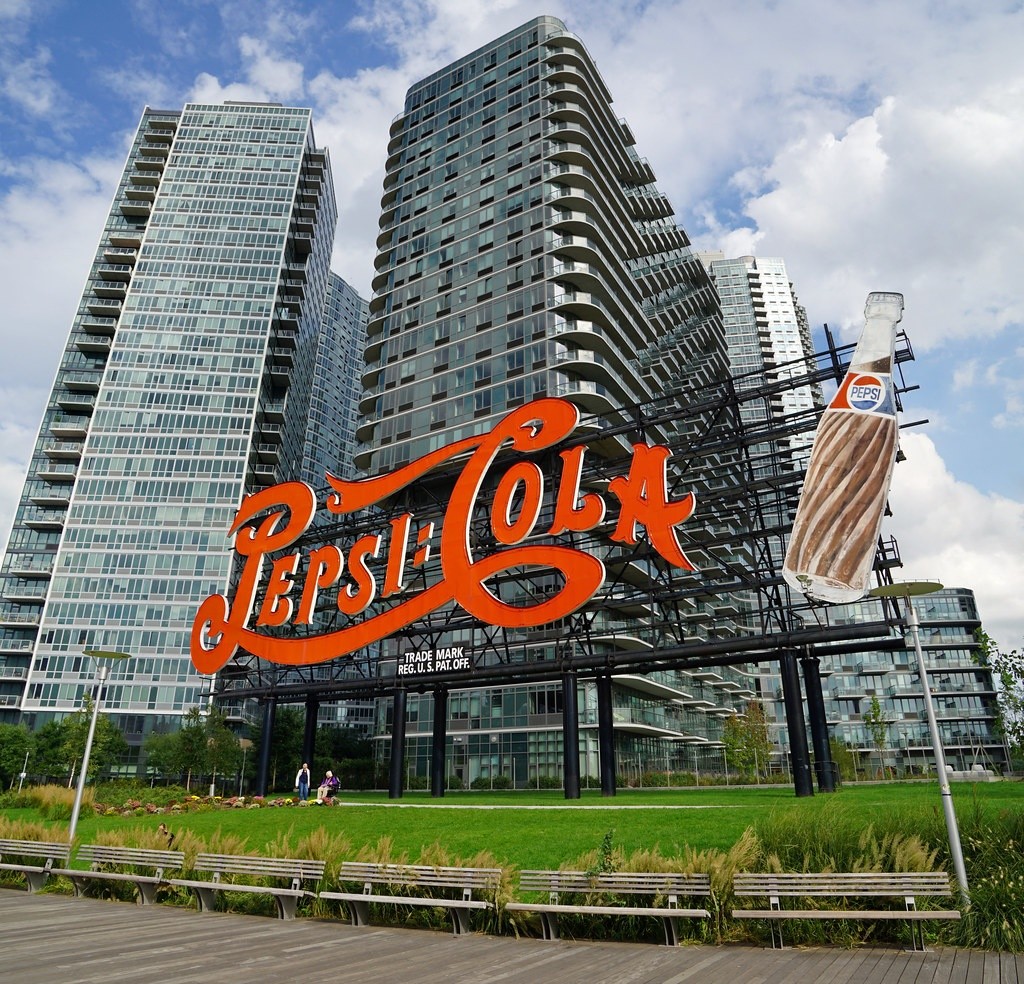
[158,824,178,852]
[295,763,311,800]
[317,770,338,798]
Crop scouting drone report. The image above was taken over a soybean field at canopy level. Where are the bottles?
[781,291,906,605]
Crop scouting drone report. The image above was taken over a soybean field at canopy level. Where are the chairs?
[50,845,185,905]
[732,872,962,952]
[170,853,326,920]
[0,838,71,892]
[319,862,501,935]
[505,870,710,946]
